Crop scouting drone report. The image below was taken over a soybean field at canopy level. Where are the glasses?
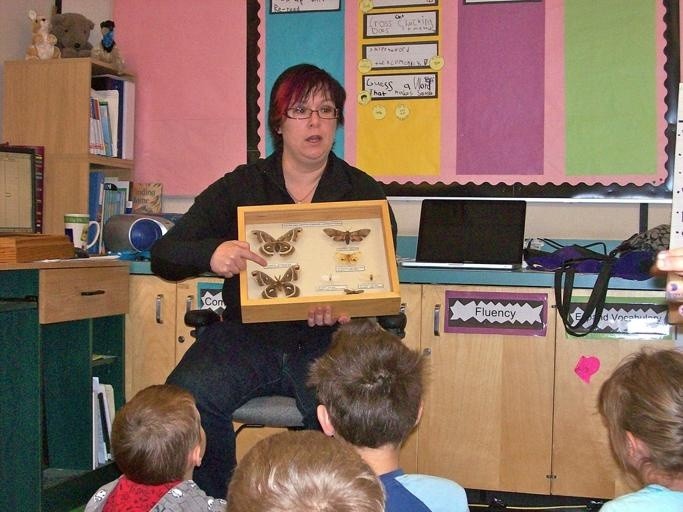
[283,105,340,120]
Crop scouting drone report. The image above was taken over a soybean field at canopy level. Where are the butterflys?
[358,272,381,283]
[251,227,303,258]
[333,251,361,268]
[323,228,371,245]
[321,272,341,283]
[251,264,300,300]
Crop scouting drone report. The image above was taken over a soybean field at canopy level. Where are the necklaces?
[283,182,320,203]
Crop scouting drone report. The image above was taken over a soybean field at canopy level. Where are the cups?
[62,211,101,252]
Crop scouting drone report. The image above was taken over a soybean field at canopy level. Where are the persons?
[309,317,470,511]
[148,63,397,500]
[221,425,387,511]
[655,246,682,277]
[81,382,224,511]
[592,347,681,511]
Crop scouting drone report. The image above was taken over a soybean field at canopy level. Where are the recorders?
[104,213,184,255]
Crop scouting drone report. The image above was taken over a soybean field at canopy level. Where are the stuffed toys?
[24,6,60,61]
[89,19,127,75]
[48,12,95,58]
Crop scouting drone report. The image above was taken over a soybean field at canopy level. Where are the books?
[88,73,135,160]
[87,172,132,255]
[90,377,118,470]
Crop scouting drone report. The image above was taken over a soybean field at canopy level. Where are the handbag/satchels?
[523,244,654,336]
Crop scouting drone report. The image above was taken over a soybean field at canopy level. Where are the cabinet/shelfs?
[552,288,683,502]
[0,265,129,512]
[126,273,289,467]
[398,283,558,497]
[0,57,136,261]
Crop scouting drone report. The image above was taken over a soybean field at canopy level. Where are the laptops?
[401,200,527,270]
